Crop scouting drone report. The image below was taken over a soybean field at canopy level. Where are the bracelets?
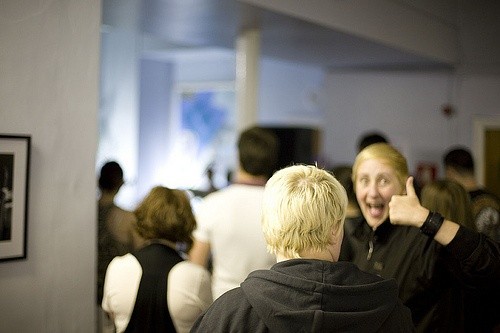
[418,209,444,240]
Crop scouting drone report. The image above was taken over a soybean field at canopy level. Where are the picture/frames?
[0,133,31,266]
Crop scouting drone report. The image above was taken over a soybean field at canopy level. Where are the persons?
[190,164,414,333]
[97,126,500,333]
